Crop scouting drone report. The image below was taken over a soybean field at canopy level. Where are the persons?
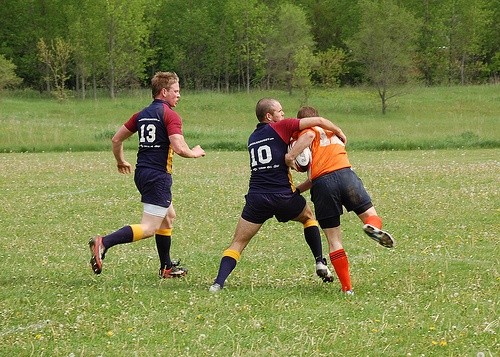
[208,97,346,294]
[285,106,394,297]
[88,71,206,279]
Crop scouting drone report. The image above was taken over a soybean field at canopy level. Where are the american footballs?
[287,136,313,173]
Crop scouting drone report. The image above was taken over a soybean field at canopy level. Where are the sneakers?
[362,224,394,248]
[157,258,189,280]
[316,258,334,283]
[341,287,355,300]
[88,236,107,274]
[209,283,227,294]
